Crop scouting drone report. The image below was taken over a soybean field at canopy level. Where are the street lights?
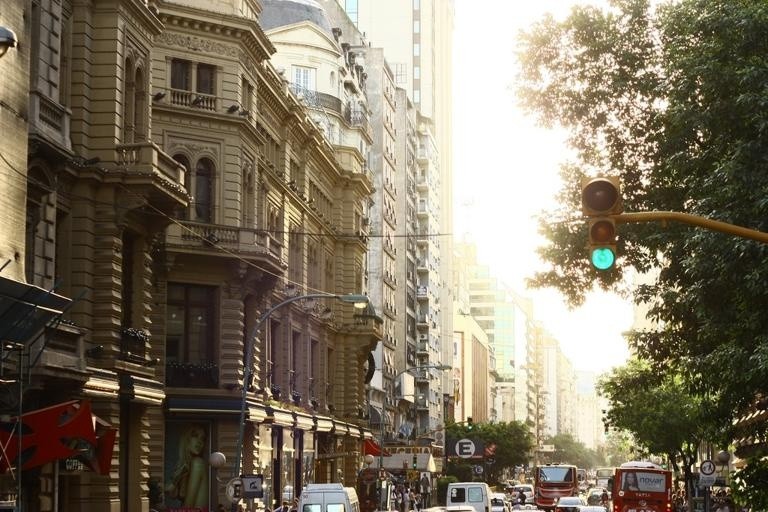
[619,330,731,512]
[363,362,455,511]
[208,291,371,511]
[0,24,17,58]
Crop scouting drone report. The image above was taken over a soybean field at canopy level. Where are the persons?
[392,471,430,511]
[164,421,208,511]
[505,488,527,506]
[216,497,299,512]
[622,472,640,491]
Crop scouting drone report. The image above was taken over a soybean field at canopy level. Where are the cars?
[444,460,686,511]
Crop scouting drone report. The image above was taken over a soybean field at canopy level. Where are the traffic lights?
[466,416,475,432]
[661,456,667,469]
[413,455,417,468]
[579,176,623,277]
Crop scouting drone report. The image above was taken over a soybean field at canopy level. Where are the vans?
[296,479,362,511]
